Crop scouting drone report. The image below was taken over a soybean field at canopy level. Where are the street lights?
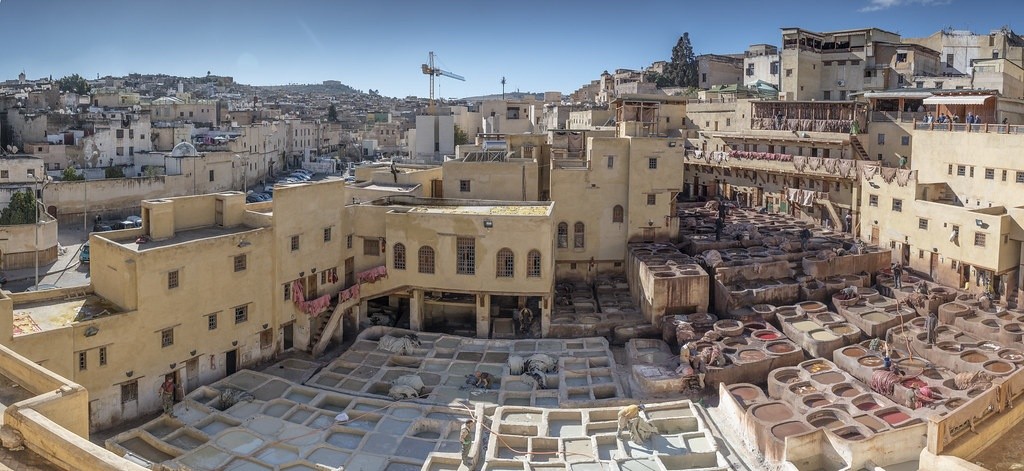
[27,173,39,292]
[84,150,98,244]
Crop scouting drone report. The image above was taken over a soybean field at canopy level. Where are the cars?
[94,223,112,231]
[79,239,90,263]
[112,220,135,230]
[126,215,142,226]
[245,166,317,206]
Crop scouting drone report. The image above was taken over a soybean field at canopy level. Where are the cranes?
[421,50,467,114]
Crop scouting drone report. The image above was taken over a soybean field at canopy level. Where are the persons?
[800,226,810,252]
[775,110,783,130]
[858,239,867,255]
[869,337,883,351]
[705,348,725,367]
[159,375,177,418]
[891,261,903,291]
[924,312,938,346]
[616,404,645,441]
[460,419,474,466]
[986,283,995,308]
[899,155,907,169]
[390,161,399,183]
[908,103,1007,134]
[519,307,533,336]
[845,213,852,234]
[715,202,726,242]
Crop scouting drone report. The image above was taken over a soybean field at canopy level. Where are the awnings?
[864,91,934,99]
[922,95,995,106]
